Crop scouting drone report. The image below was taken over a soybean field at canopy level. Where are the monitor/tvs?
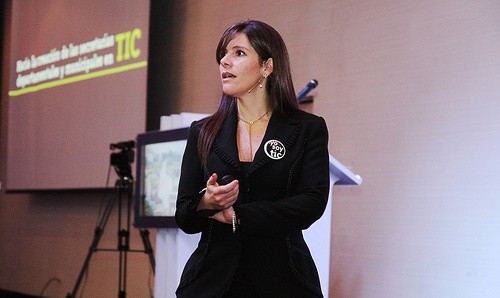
[133,128,192,227]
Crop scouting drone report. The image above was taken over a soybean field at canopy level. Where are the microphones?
[293,79,317,101]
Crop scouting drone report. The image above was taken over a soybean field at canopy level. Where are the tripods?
[65,176,155,298]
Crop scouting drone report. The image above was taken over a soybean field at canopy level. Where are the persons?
[175,20,330,298]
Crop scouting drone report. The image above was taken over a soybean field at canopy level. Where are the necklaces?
[237,111,270,160]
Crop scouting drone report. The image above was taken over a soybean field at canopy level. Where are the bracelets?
[230,212,238,236]
